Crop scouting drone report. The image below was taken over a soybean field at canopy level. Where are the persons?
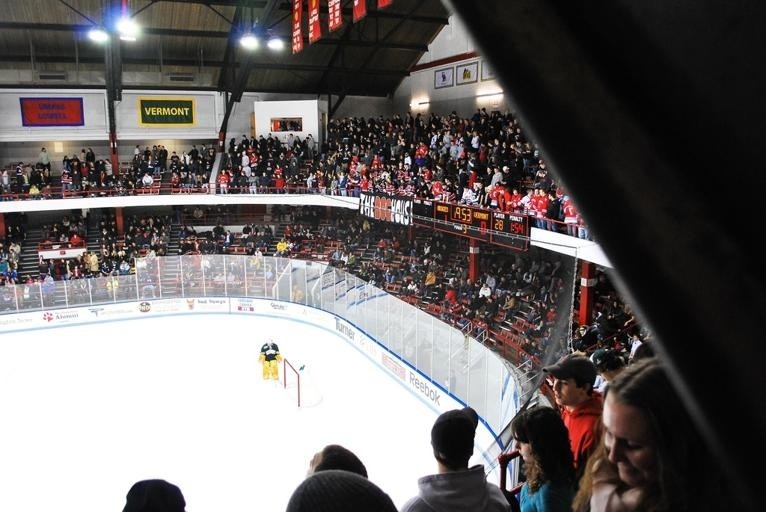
[1,107,655,392]
[286,351,739,512]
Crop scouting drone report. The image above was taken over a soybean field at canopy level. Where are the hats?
[589,348,619,366]
[432,407,478,448]
[543,349,596,379]
[286,470,399,512]
